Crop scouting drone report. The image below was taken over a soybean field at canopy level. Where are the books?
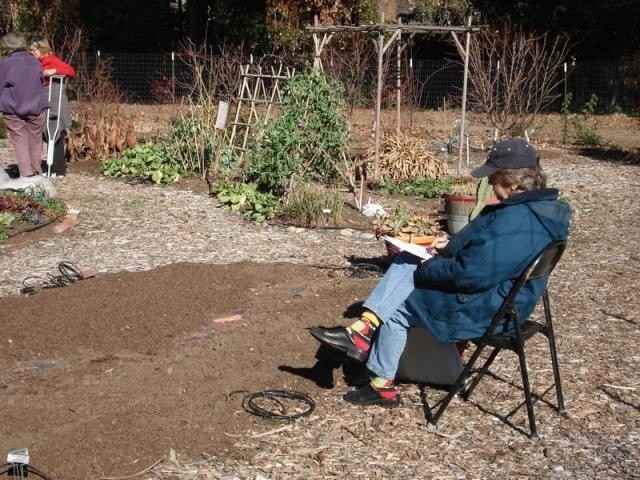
[380,234,433,261]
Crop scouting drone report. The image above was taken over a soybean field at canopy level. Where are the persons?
[1,32,52,180]
[28,38,77,181]
[308,135,573,410]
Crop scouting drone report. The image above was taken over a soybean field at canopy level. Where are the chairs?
[421,235,568,439]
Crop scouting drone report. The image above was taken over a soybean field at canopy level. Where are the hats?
[469,139,539,177]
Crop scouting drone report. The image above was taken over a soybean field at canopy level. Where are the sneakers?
[42,171,65,180]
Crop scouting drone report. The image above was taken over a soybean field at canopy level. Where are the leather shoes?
[310,324,368,362]
[344,385,401,409]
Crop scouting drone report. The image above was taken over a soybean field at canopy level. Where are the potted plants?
[374,205,440,260]
[441,178,478,236]
